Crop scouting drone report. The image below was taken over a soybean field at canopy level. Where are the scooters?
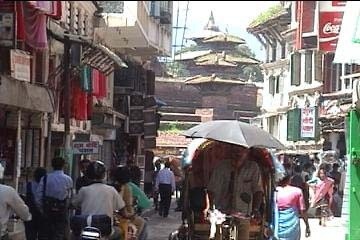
[68,202,125,240]
[114,190,158,239]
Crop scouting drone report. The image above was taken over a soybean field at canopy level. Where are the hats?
[332,163,340,167]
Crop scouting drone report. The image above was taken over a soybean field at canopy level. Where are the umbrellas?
[179,119,292,150]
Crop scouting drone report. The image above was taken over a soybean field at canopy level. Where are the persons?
[274,169,311,240]
[76,158,185,240]
[0,164,32,240]
[36,155,74,240]
[23,166,48,240]
[285,158,343,227]
[203,144,266,240]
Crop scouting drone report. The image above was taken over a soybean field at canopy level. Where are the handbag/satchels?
[43,197,66,218]
[70,215,112,236]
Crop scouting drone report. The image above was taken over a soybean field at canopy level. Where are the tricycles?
[171,138,276,240]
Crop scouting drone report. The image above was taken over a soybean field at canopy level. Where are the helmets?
[86,160,105,179]
